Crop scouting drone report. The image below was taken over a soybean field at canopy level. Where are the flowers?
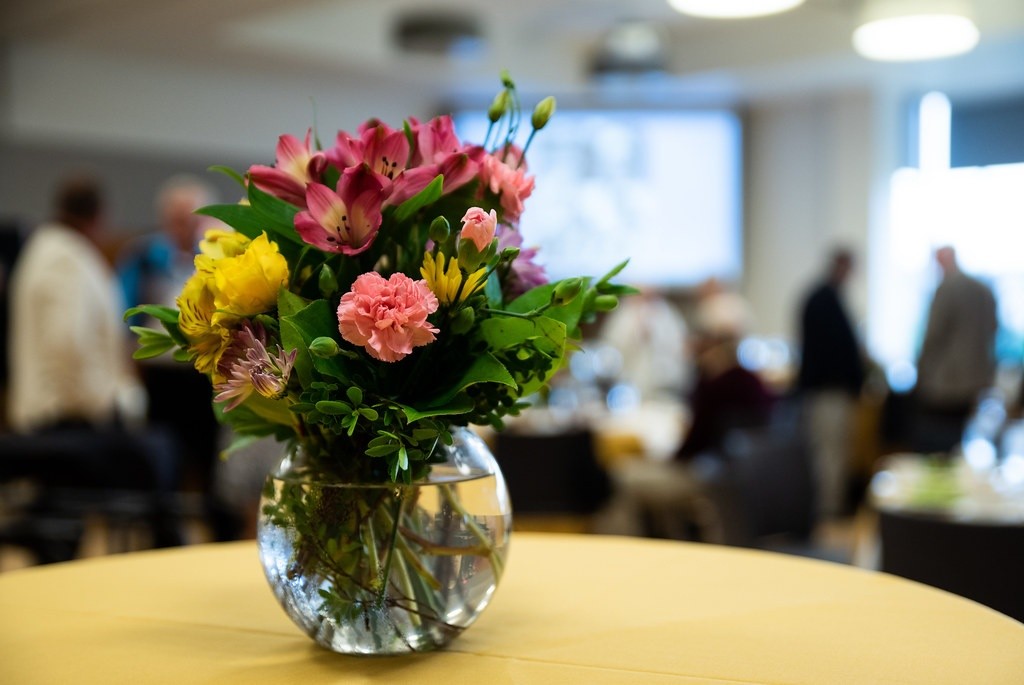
[122,73,640,483]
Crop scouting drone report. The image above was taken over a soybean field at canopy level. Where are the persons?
[492,237,1024,537]
[9,168,246,566]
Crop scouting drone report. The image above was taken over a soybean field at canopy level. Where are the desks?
[0,528,1023,685]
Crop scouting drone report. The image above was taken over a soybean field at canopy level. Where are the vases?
[256,427,513,655]
